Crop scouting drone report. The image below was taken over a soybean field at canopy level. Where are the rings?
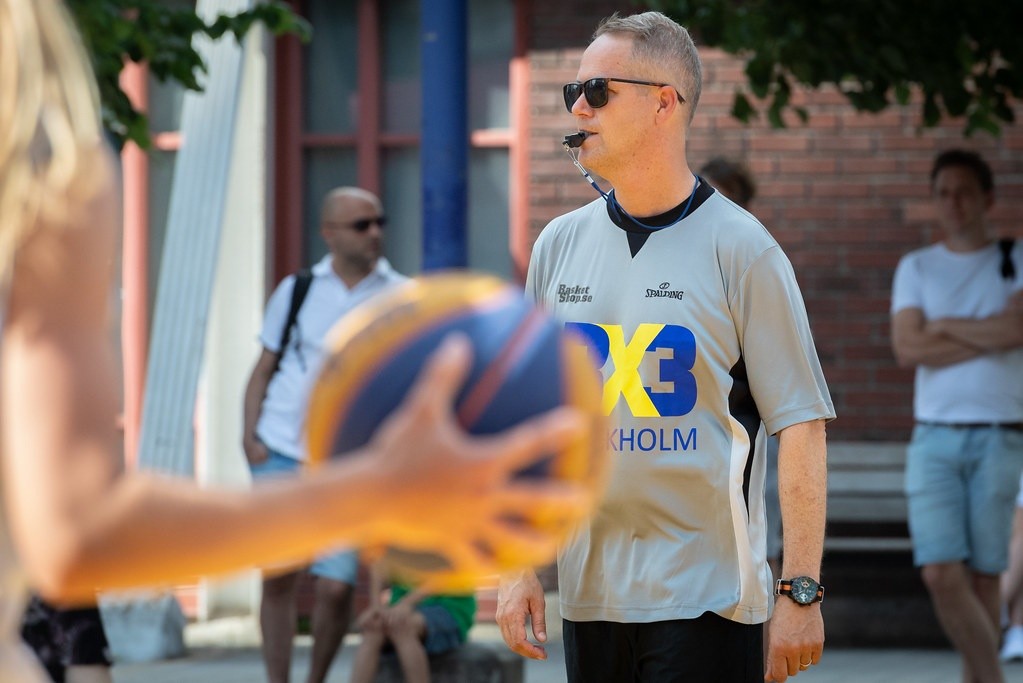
[800,660,812,666]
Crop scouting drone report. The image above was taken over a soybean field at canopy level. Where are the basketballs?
[297,271,611,596]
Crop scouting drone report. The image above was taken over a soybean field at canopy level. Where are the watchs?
[773,576,826,607]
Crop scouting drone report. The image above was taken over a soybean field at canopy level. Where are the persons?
[491,11,837,682]
[891,145,1023,683]
[0,1,594,683]
[351,528,476,682]
[243,180,411,683]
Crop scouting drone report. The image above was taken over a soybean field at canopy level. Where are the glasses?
[326,217,387,232]
[563,78,686,112]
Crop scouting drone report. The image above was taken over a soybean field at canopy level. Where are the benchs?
[779,440,912,549]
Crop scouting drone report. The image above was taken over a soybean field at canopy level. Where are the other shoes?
[1002,624,1023,661]
